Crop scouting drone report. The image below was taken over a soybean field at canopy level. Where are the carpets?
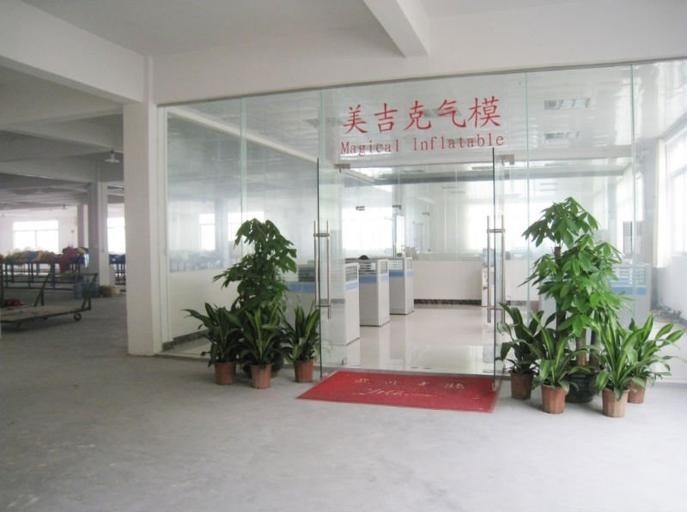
[296,368,498,414]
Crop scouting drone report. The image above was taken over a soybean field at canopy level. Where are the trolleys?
[0,269,98,328]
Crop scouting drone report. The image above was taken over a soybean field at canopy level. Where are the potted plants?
[178,218,322,390]
[493,294,540,400]
[611,318,683,405]
[530,323,574,414]
[541,195,616,403]
[586,318,638,417]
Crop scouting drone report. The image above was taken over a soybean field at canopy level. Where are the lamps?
[103,151,120,164]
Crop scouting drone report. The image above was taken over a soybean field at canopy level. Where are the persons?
[357,254,374,274]
